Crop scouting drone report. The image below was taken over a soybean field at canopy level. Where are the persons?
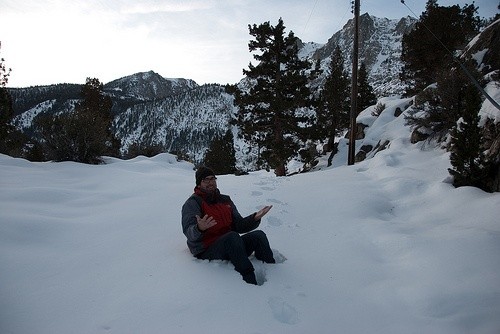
[181,166,276,285]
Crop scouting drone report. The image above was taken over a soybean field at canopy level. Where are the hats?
[195,167,215,186]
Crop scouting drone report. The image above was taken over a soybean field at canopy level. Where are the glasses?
[202,177,217,183]
